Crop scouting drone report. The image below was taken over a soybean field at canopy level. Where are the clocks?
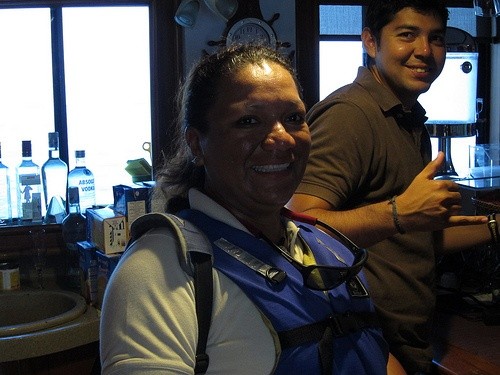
[206,0,291,52]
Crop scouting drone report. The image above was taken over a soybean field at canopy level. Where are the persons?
[100,43,405,375]
[283,0,500,375]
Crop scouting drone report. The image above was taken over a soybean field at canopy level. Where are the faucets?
[27,229,45,272]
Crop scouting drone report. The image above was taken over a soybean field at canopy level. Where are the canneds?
[0,263,20,290]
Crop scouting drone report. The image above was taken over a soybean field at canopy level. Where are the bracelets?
[489,213,499,239]
[389,195,406,235]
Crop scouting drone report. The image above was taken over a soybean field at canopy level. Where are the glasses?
[232,206,369,291]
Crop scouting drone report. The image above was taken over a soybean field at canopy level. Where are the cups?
[28,228,47,271]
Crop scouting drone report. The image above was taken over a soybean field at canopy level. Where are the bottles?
[434,122,459,180]
[42,131,68,211]
[0,142,13,226]
[62,186,90,269]
[67,150,96,215]
[16,140,43,219]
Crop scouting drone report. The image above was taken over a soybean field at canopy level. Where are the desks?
[455,177,500,217]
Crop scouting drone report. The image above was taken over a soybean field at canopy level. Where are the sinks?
[0,290,86,337]
